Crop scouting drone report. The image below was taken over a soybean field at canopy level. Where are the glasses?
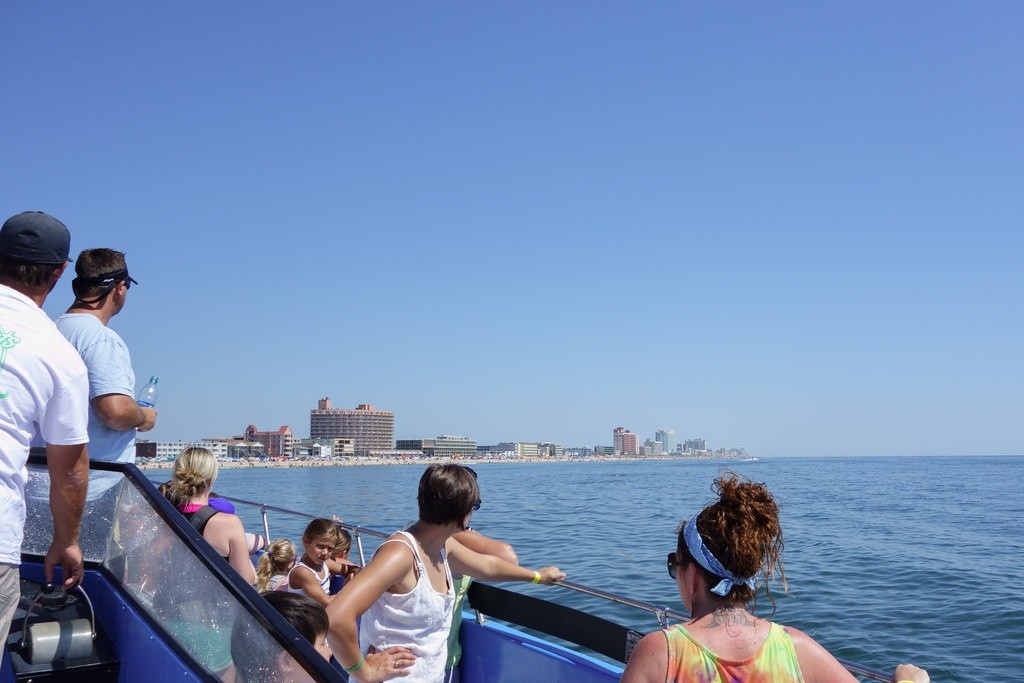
[667,552,690,580]
[124,276,132,290]
[473,497,482,511]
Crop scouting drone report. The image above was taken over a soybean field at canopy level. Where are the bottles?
[136,373,159,406]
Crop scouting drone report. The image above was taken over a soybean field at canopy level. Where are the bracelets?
[529,570,541,584]
[344,651,365,673]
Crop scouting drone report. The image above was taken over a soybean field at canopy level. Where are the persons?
[1,209,88,675]
[248,530,296,593]
[621,474,929,682]
[24,247,158,467]
[280,518,360,614]
[157,446,254,587]
[322,461,564,682]
[326,528,361,590]
[438,464,518,683]
[214,590,332,681]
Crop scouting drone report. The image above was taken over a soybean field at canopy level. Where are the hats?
[0,210,73,264]
